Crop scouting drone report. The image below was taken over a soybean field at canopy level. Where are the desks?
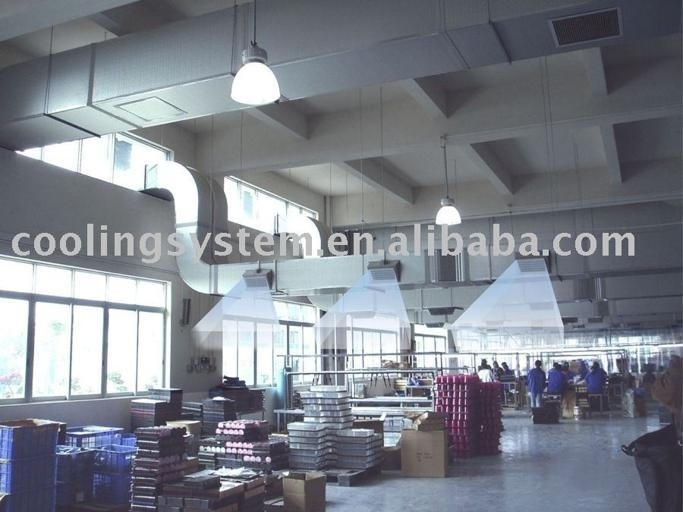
[502,375,642,413]
[267,395,434,430]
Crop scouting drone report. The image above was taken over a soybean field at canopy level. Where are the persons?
[500,361,512,391]
[526,359,546,407]
[617,354,682,511]
[478,364,494,382]
[491,361,505,380]
[547,357,654,409]
[477,358,491,371]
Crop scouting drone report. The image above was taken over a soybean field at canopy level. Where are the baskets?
[0,418,137,511]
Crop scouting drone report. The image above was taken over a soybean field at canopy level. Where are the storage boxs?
[281,473,326,512]
[400,429,447,478]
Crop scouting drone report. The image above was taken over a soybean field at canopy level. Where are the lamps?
[434,132,462,226]
[230,0,280,108]
